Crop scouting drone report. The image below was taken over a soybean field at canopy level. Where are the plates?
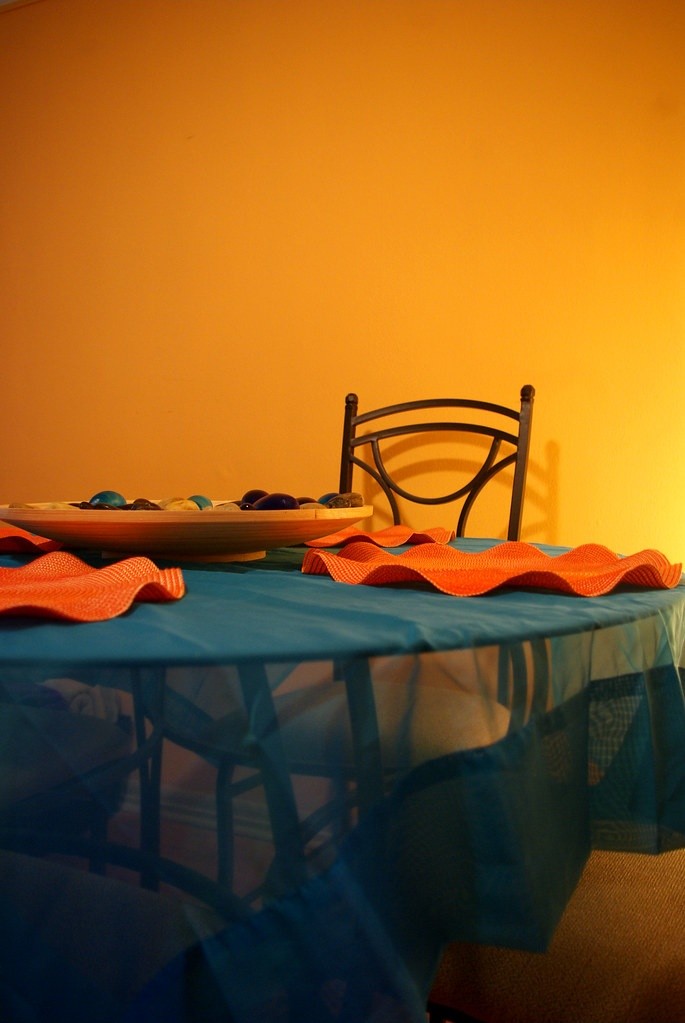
[1,500,373,563]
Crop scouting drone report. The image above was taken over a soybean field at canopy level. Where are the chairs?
[0,384,539,1023]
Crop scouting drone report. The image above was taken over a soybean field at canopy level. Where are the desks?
[0,541,684,1022]
